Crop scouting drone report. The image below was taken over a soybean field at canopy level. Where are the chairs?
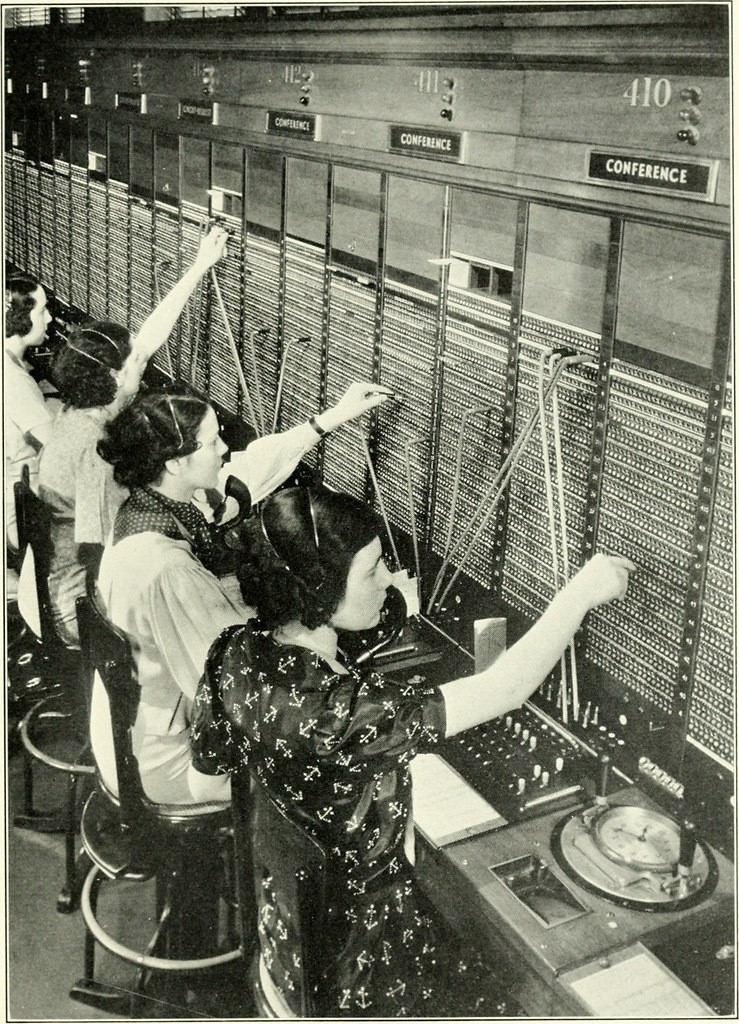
[14,481,97,831]
[69,569,246,1018]
[229,767,330,1018]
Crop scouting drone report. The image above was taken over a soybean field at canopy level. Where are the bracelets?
[309,416,334,438]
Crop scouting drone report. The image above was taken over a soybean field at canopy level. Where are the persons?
[86,382,395,817]
[5,273,58,599]
[189,478,638,1017]
[19,226,230,649]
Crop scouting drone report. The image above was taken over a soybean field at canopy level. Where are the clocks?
[593,806,680,871]
